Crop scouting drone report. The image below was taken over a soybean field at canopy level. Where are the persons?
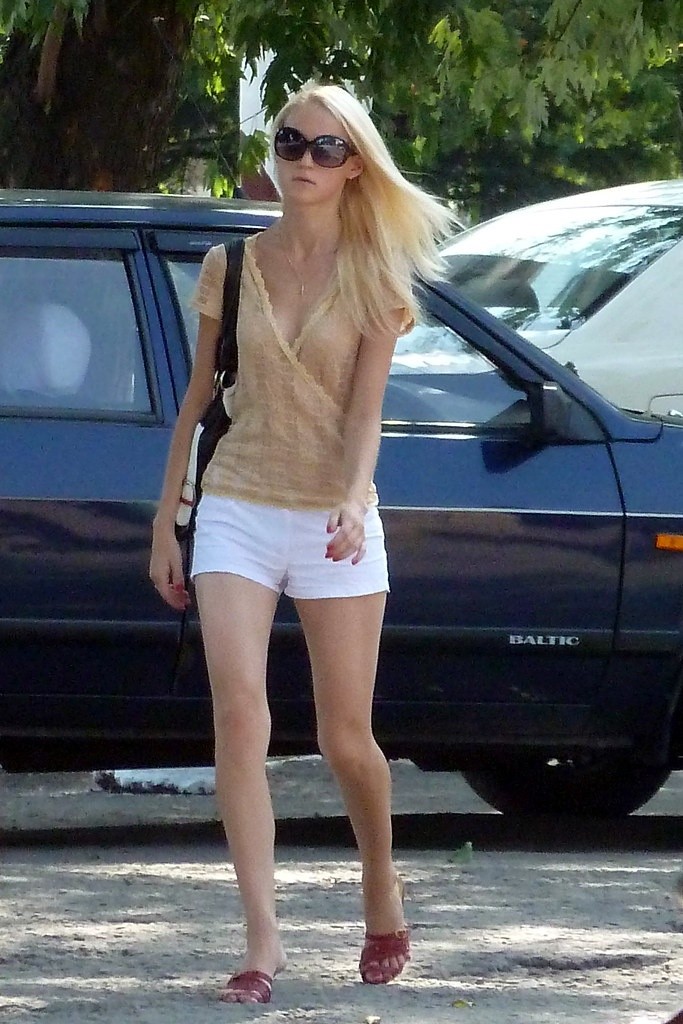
[147,85,463,1004]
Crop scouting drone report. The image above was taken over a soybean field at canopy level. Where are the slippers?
[215,967,274,1008]
[359,877,410,985]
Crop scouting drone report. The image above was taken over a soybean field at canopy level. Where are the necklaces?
[278,233,323,297]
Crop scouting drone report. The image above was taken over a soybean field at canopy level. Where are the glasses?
[274,127,356,169]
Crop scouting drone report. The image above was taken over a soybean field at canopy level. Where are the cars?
[399,179,683,410]
[0,190,683,833]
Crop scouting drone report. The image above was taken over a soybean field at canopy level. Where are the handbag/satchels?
[174,232,247,581]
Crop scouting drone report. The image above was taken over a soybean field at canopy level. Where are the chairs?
[3,299,93,407]
